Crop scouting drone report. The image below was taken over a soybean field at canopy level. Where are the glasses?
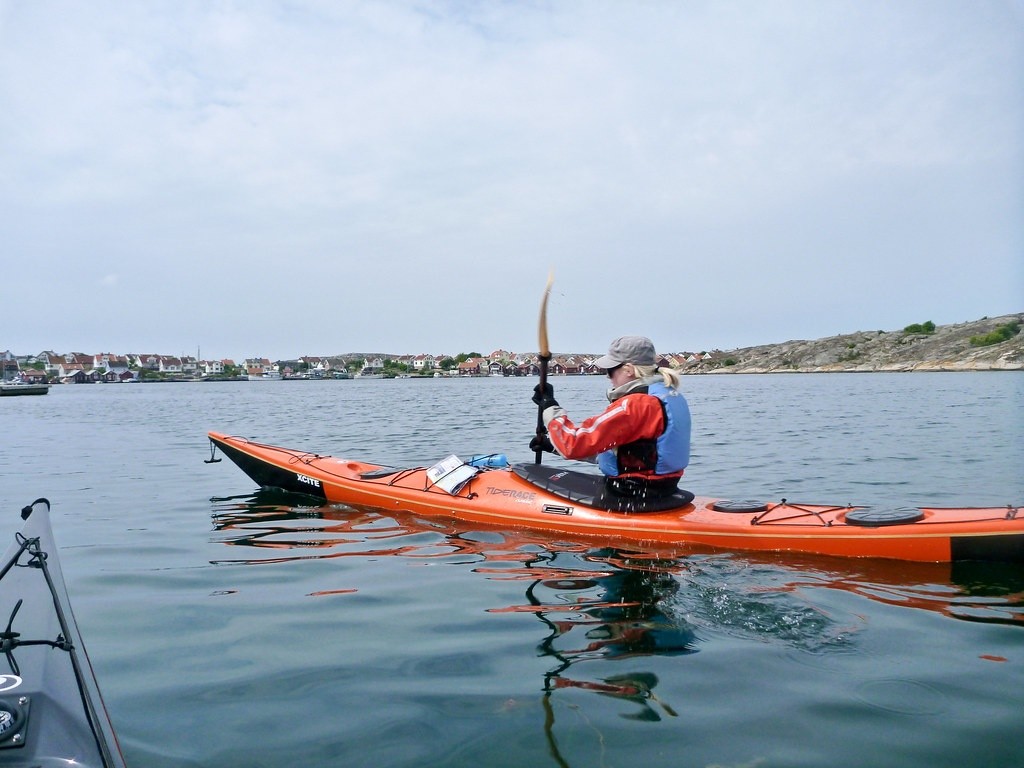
[607,364,624,378]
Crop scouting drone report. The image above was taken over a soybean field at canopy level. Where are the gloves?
[532,383,559,411]
[529,436,556,453]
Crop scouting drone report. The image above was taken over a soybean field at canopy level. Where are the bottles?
[464,455,507,466]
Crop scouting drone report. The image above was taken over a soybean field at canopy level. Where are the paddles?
[534,270,556,465]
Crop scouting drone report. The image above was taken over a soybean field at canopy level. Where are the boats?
[208,433,1024,567]
[0,499,125,768]
[7,377,27,384]
[0,384,52,395]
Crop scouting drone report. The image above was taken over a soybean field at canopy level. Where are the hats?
[594,335,656,370]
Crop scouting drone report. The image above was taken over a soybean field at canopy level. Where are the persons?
[531,334,692,509]
